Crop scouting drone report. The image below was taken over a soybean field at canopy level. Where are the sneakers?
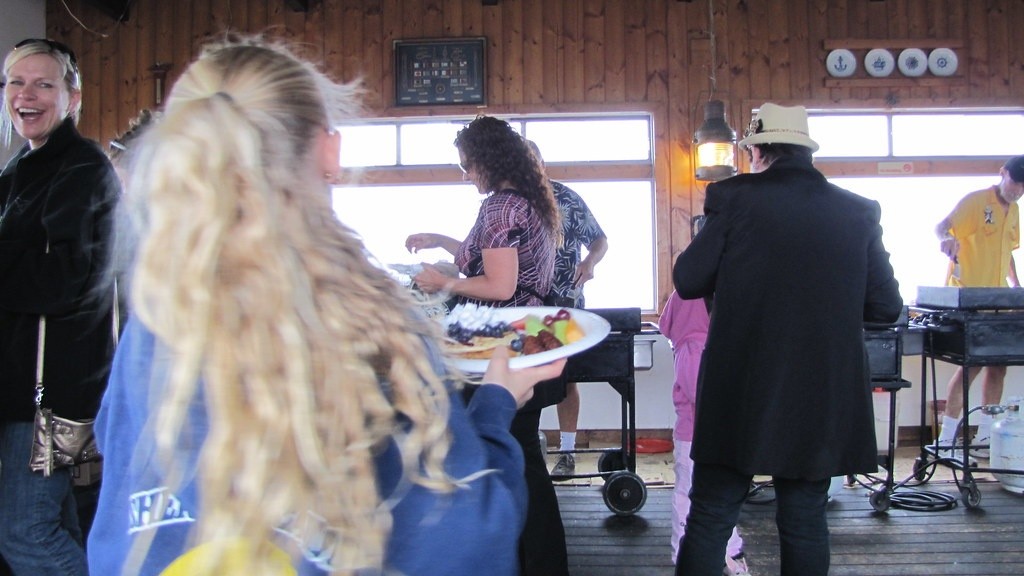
[549,455,576,480]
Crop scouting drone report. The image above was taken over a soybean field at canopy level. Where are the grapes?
[544,310,571,326]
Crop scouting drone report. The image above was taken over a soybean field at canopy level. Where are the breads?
[438,323,523,359]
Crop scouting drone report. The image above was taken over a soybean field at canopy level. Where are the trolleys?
[846,324,911,512]
[913,309,1024,511]
[546,306,664,517]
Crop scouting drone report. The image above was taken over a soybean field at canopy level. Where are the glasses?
[13,38,77,69]
[459,161,473,175]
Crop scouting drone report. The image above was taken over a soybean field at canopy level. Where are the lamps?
[693,0,739,181]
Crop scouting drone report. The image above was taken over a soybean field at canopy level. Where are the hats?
[736,104,820,152]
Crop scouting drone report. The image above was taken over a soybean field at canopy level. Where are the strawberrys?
[523,329,563,355]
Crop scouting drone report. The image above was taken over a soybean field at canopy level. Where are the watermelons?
[509,317,525,329]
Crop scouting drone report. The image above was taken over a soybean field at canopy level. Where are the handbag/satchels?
[29,409,103,477]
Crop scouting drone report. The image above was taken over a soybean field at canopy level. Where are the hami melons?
[564,319,585,344]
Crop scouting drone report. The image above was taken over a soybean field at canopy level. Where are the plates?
[441,307,612,372]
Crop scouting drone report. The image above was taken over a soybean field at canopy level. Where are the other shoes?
[723,551,752,576]
[968,436,992,458]
[934,438,978,468]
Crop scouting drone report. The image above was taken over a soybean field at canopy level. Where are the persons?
[660,217,748,576]
[672,102,904,576]
[405,115,580,576]
[521,137,608,480]
[0,38,123,576]
[86,35,567,576]
[933,154,1024,470]
